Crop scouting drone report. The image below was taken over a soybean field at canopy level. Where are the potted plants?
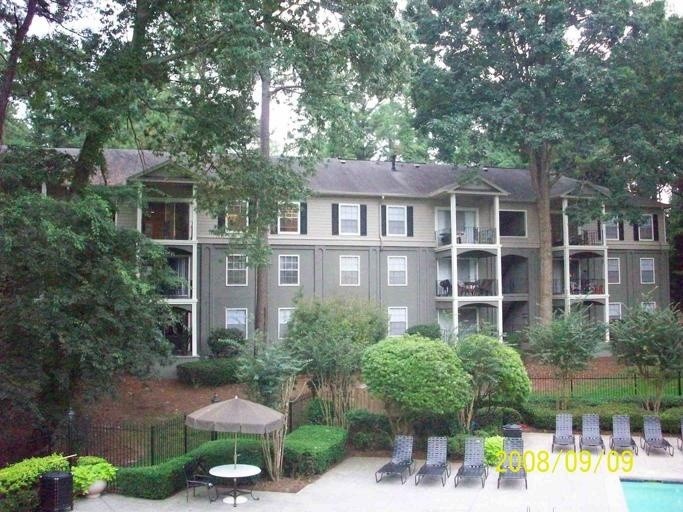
[70,455,119,498]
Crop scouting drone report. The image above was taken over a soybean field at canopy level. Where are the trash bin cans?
[38,471,73,512]
[501,423,523,437]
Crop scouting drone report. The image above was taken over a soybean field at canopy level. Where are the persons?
[583,282,595,295]
[571,281,579,294]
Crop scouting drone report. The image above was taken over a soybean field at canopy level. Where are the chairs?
[454,436,489,488]
[640,415,674,456]
[579,414,605,454]
[182,458,219,504]
[375,435,417,485]
[438,227,494,296]
[551,414,576,454]
[556,231,603,294]
[677,417,683,451]
[609,415,639,455]
[497,437,527,489]
[414,436,451,486]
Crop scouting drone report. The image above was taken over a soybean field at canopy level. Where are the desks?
[209,464,261,507]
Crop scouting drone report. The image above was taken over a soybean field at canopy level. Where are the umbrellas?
[185,395,284,498]
